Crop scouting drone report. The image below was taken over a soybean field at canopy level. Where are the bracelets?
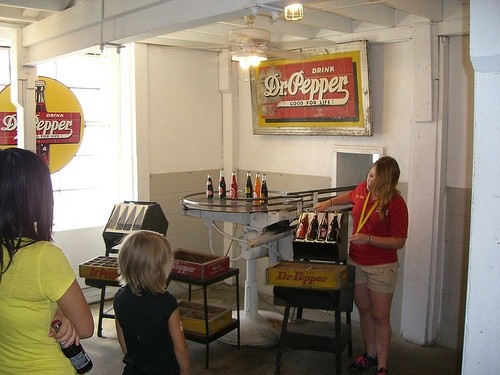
[328,198,332,207]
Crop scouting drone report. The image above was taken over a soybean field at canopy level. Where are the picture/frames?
[251,41,372,135]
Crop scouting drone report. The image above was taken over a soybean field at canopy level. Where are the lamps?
[228,3,303,70]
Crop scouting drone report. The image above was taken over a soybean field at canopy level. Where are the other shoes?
[348,352,391,375]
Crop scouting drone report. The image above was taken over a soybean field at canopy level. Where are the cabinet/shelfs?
[272,211,359,375]
[167,267,242,371]
[85,278,125,336]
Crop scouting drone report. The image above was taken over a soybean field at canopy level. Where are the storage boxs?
[265,261,348,290]
[174,301,233,335]
[169,247,231,280]
[77,257,122,280]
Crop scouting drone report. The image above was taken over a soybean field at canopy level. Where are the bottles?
[327,215,339,241]
[319,210,328,240]
[245,172,253,202]
[51,319,92,374]
[254,172,268,207]
[206,174,213,198]
[231,171,238,201]
[219,170,226,200]
[296,213,308,239]
[307,215,318,240]
[35,79,49,167]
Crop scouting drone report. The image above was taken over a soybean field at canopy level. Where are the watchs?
[368,234,372,245]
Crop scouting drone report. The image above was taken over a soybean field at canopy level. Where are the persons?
[113,230,191,375]
[313,155,408,375]
[0,147,95,375]
